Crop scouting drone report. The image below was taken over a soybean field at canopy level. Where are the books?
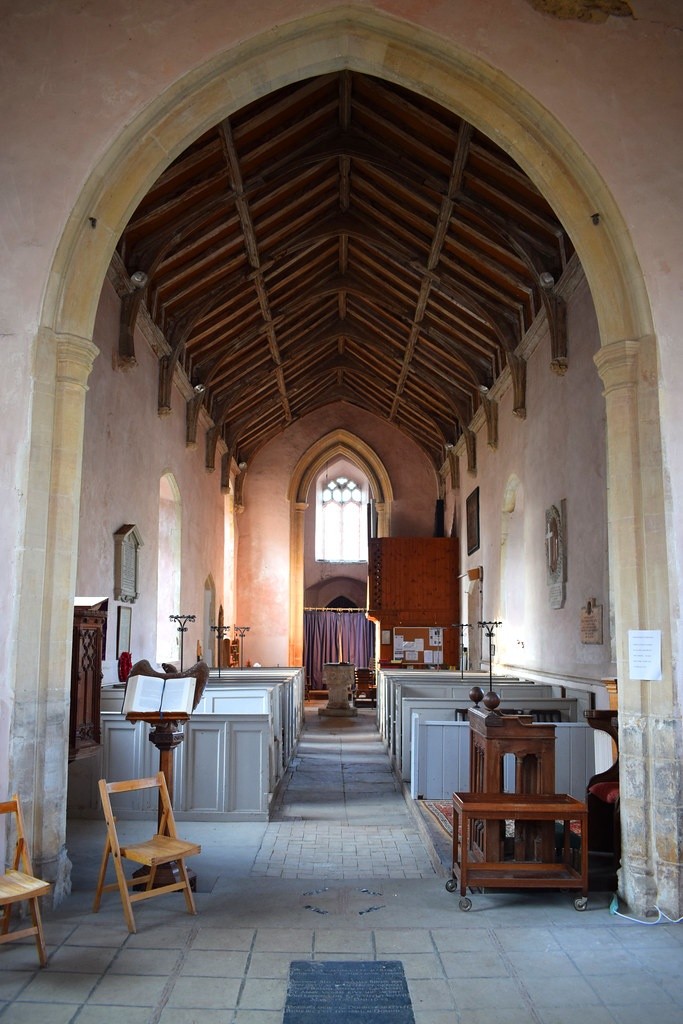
[121,674,197,715]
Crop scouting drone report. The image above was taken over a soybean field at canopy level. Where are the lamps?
[190,375,206,394]
[127,264,148,288]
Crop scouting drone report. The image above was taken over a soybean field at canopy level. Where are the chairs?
[94,770,203,934]
[0,792,52,969]
[352,669,376,710]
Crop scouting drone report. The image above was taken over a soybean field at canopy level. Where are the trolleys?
[445,790,589,912]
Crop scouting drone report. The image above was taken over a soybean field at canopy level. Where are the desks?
[355,666,372,699]
